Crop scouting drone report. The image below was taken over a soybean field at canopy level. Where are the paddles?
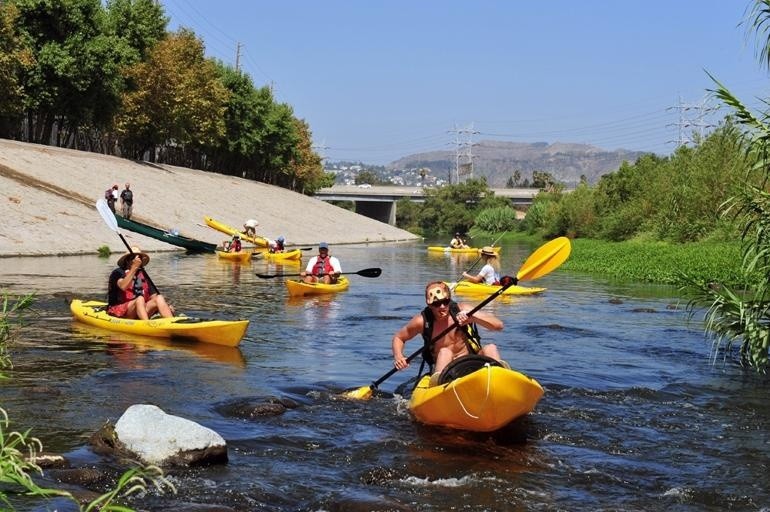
[347,265,525,402]
[255,268,383,279]
[94,198,161,294]
[252,247,312,258]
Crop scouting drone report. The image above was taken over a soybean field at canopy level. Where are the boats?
[261,248,303,261]
[111,212,220,258]
[69,295,252,350]
[285,271,350,297]
[440,280,550,296]
[410,352,544,435]
[204,215,269,248]
[214,249,252,263]
[427,245,502,256]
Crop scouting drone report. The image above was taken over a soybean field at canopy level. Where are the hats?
[318,242,329,251]
[477,246,499,257]
[117,247,150,267]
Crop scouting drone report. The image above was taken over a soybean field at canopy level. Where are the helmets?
[424,281,451,305]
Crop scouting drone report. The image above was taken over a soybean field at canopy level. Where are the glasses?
[430,298,450,307]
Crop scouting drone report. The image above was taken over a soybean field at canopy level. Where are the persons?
[461,245,501,285]
[243,223,258,245]
[228,235,241,253]
[299,242,342,284]
[120,183,133,220]
[105,185,117,214]
[106,245,175,320]
[391,280,504,388]
[450,232,470,249]
[265,236,285,253]
